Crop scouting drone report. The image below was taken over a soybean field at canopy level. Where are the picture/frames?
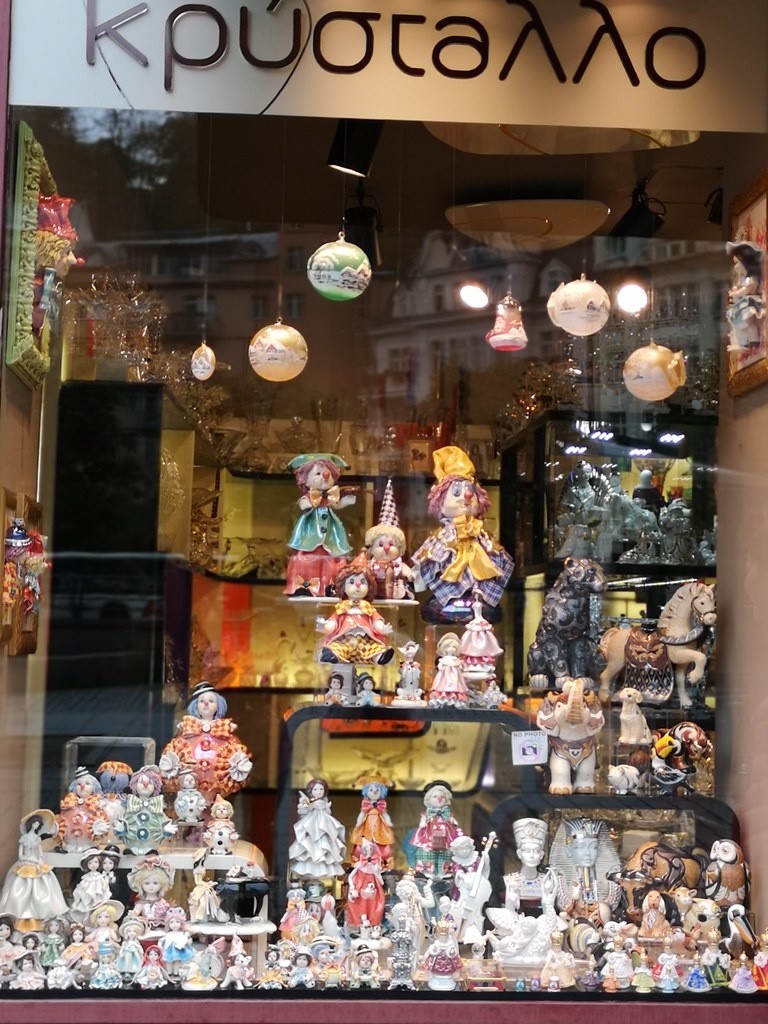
[6,120,84,392]
[725,174,768,400]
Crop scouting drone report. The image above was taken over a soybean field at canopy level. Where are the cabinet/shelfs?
[191,444,529,911]
[498,410,720,733]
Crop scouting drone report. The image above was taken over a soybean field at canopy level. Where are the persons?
[32,196,86,334]
[0,686,256,990]
[286,446,506,707]
[560,460,594,526]
[725,246,765,349]
[256,779,767,994]
[631,468,667,530]
[1,524,53,655]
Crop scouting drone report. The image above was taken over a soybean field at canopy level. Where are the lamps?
[342,179,382,267]
[704,187,722,225]
[327,118,386,178]
[604,176,666,266]
